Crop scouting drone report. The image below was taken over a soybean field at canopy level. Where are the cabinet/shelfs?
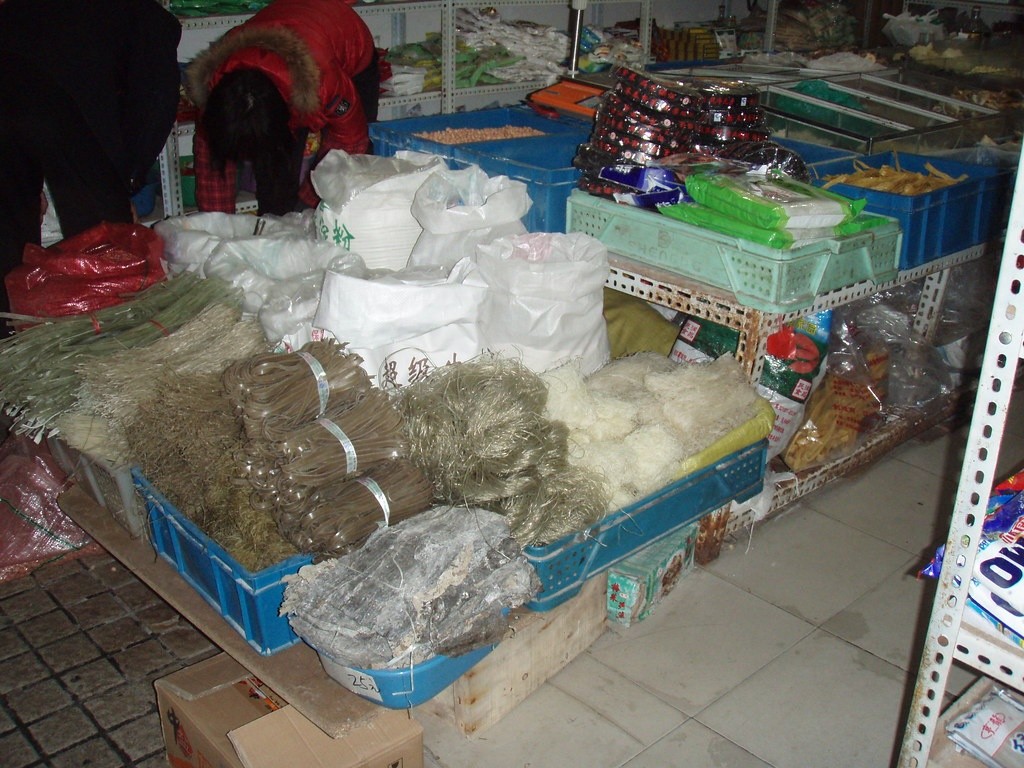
[897,143,1024,768]
[154,0,977,222]
[891,137,1024,768]
[604,242,998,565]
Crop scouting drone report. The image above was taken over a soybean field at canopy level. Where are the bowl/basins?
[295,608,511,710]
[149,154,242,206]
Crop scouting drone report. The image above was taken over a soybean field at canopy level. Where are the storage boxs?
[42,106,1016,768]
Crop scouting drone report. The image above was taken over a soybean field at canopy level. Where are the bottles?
[966,7,983,46]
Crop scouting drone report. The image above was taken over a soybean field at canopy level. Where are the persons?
[0,0,182,339]
[185,0,381,216]
